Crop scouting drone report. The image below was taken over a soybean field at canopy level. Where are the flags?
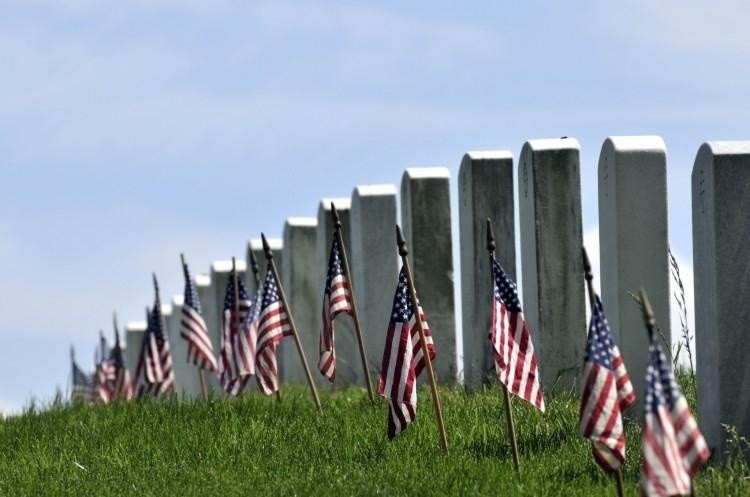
[71,255,294,409]
[316,233,356,384]
[485,260,545,415]
[641,320,713,495]
[578,293,638,477]
[374,263,440,440]
